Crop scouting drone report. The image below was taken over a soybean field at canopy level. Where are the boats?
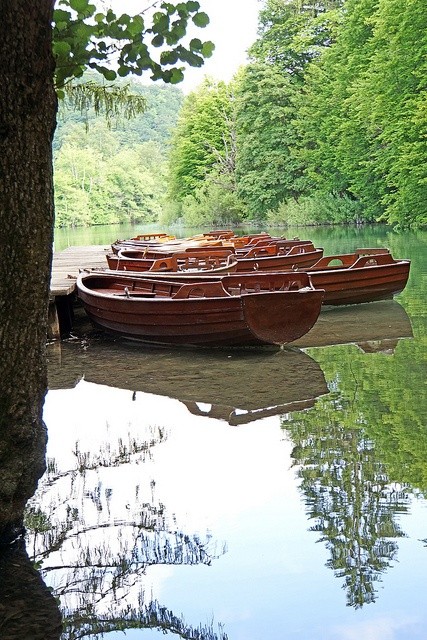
[83,338,331,427]
[66,233,196,278]
[197,248,324,273]
[284,299,415,356]
[197,247,411,305]
[76,274,325,346]
[196,230,313,246]
